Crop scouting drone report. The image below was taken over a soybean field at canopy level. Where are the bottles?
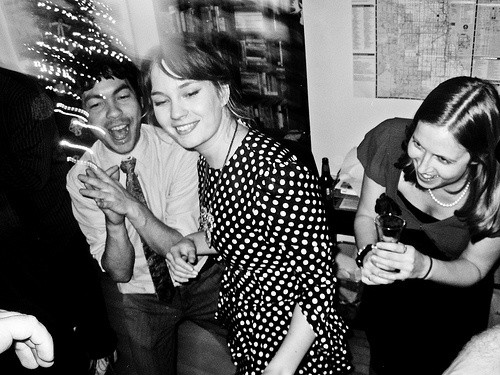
[320,157,334,205]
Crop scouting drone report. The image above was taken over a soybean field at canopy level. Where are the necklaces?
[429,181,470,208]
[201,119,240,249]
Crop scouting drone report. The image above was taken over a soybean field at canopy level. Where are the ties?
[120,157,175,304]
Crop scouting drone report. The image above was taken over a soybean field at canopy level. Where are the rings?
[368,273,373,281]
[402,245,407,254]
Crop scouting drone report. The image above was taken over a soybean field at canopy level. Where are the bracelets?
[355,244,378,268]
[420,257,433,280]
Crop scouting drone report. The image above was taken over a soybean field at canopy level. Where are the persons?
[354,76,500,375]
[141,37,354,375]
[62,47,227,375]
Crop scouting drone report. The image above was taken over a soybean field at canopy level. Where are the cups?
[375,213,406,243]
